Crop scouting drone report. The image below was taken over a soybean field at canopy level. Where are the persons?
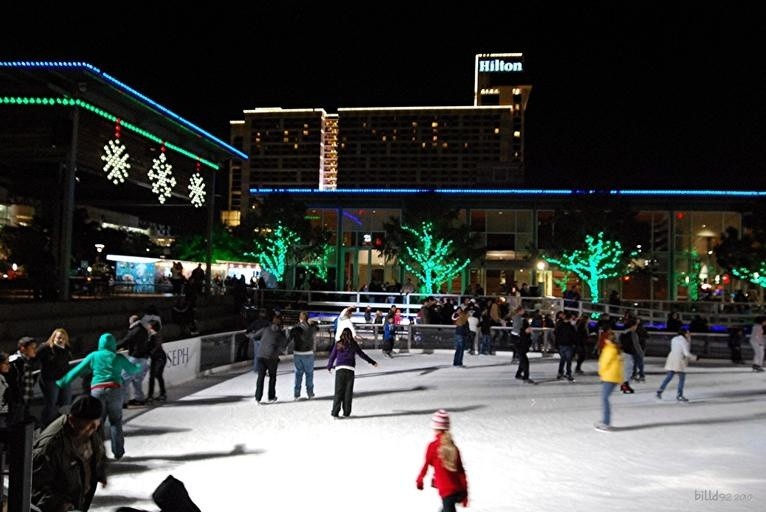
[0,314,166,512]
[215,271,765,429]
[416,408,468,512]
[170,262,205,336]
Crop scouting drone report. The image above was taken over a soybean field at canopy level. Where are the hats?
[431,409,450,430]
[58,395,102,419]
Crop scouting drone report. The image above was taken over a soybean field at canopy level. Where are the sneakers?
[594,423,609,432]
[620,384,632,391]
[514,368,582,385]
[656,391,688,401]
